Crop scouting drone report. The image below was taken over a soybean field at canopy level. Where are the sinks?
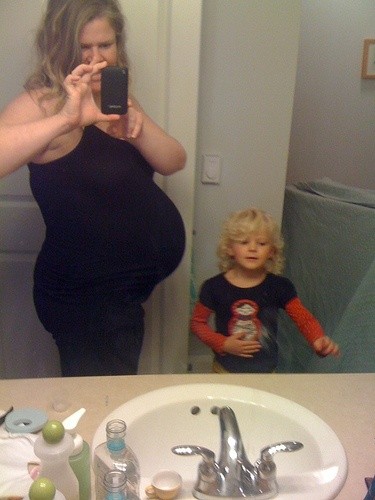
[91,383,348,499]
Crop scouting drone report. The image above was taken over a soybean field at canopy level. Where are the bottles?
[103,469,129,500]
[93,419,140,500]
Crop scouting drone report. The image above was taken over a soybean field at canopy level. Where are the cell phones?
[101,67,127,115]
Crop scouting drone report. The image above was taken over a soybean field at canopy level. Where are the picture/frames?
[361,38,375,79]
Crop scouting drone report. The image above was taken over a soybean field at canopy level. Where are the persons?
[188,208,341,373]
[0,0,188,377]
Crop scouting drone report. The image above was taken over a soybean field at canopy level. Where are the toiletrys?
[33,406,91,500]
[24,478,56,500]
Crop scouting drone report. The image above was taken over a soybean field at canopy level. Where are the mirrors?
[1,0,375,380]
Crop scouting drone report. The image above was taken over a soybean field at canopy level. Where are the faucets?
[171,405,304,499]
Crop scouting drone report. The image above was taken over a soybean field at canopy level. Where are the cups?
[146,470,182,500]
